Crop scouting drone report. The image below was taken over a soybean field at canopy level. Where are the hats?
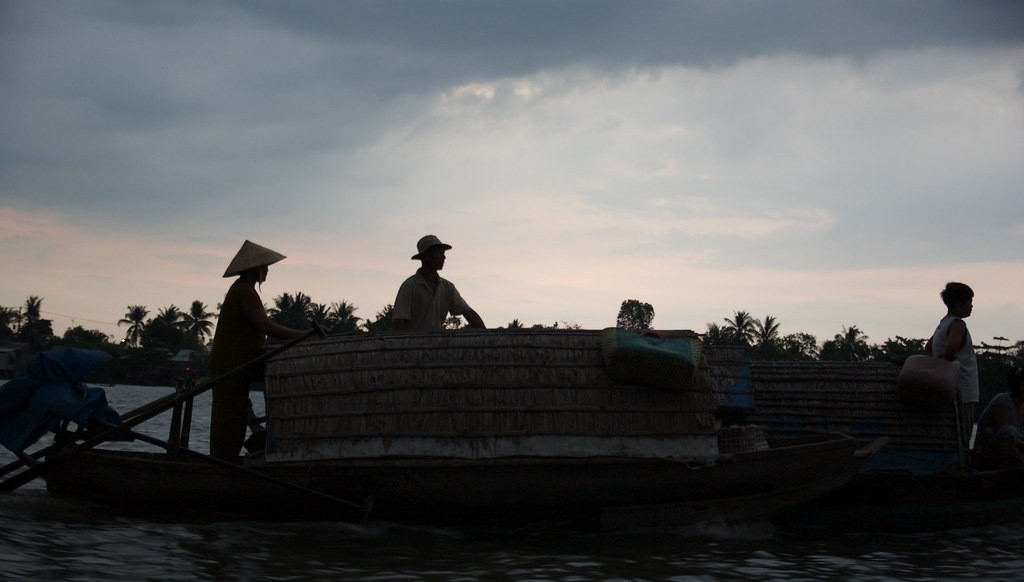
[411,235,452,259]
[224,240,286,278]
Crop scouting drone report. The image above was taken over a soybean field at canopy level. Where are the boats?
[48,329,1024,540]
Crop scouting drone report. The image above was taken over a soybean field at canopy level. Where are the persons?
[210,241,305,457]
[926,283,980,404]
[392,236,486,329]
[975,367,1024,467]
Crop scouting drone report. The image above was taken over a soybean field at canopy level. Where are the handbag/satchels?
[895,355,960,410]
[600,327,701,390]
